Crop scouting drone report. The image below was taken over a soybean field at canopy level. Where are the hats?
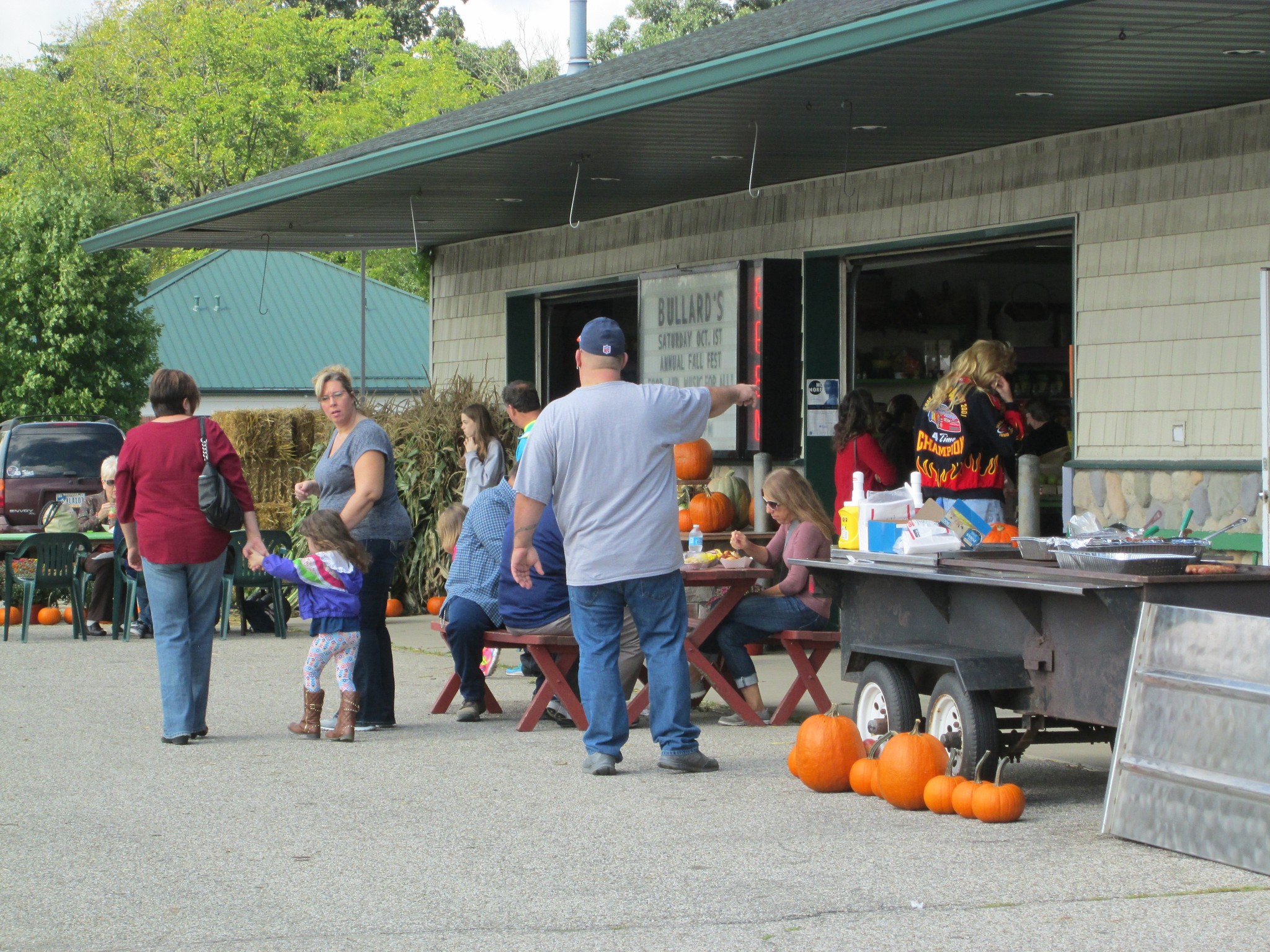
[577,317,625,357]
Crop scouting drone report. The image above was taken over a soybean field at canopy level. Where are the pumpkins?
[678,487,733,533]
[972,757,1025,822]
[427,583,446,615]
[99,606,141,624]
[0,604,21,625]
[749,497,781,531]
[981,523,1019,548]
[952,751,993,819]
[924,748,967,814]
[64,607,87,625]
[788,703,949,812]
[707,470,752,531]
[37,592,61,625]
[674,438,714,480]
[385,592,403,617]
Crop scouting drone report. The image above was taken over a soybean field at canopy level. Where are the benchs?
[429,622,588,733]
[685,616,842,726]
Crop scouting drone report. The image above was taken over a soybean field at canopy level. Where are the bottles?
[838,500,859,550]
[108,506,117,530]
[688,524,703,552]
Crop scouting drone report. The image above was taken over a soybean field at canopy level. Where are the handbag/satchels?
[38,500,79,533]
[197,416,244,529]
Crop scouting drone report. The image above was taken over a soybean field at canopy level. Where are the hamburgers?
[722,551,740,560]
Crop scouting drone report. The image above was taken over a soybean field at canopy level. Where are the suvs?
[0,414,128,553]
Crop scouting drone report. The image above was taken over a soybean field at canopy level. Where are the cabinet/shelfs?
[676,479,777,549]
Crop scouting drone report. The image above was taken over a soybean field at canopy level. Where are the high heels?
[161,734,189,745]
[192,725,209,740]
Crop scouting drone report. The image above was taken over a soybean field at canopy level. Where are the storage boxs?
[867,519,938,554]
[941,498,993,551]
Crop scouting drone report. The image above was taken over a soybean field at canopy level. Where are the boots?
[325,691,359,742]
[288,686,323,740]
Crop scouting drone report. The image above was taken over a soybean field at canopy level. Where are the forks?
[735,530,738,555]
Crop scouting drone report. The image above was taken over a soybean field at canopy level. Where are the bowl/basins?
[679,551,719,571]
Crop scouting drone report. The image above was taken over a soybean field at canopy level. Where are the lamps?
[422,246,435,261]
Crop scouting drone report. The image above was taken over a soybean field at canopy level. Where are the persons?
[884,393,919,490]
[114,369,269,743]
[295,365,413,730]
[248,510,371,742]
[502,381,542,675]
[439,458,553,720]
[833,387,898,535]
[113,518,154,638]
[916,340,1024,524]
[437,504,500,677]
[1019,395,1066,537]
[688,466,835,725]
[498,493,645,727]
[511,317,760,775]
[461,404,504,508]
[77,456,137,636]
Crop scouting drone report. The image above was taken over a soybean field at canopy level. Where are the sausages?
[1185,564,1236,574]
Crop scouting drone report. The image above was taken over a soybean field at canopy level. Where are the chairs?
[2,529,292,643]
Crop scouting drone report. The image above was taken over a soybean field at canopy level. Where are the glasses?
[761,494,783,511]
[320,388,349,402]
[103,479,115,487]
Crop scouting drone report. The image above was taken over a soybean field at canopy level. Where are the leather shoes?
[111,624,123,633]
[85,622,107,636]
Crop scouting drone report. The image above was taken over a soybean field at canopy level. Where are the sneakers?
[531,696,551,720]
[456,700,485,721]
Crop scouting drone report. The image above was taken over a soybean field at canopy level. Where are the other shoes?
[718,706,771,727]
[120,619,146,639]
[689,679,706,700]
[143,624,155,638]
[582,750,616,776]
[657,749,719,772]
[547,700,577,728]
[376,722,393,728]
[319,717,376,731]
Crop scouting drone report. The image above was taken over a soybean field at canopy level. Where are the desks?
[0,533,113,547]
[626,560,773,725]
